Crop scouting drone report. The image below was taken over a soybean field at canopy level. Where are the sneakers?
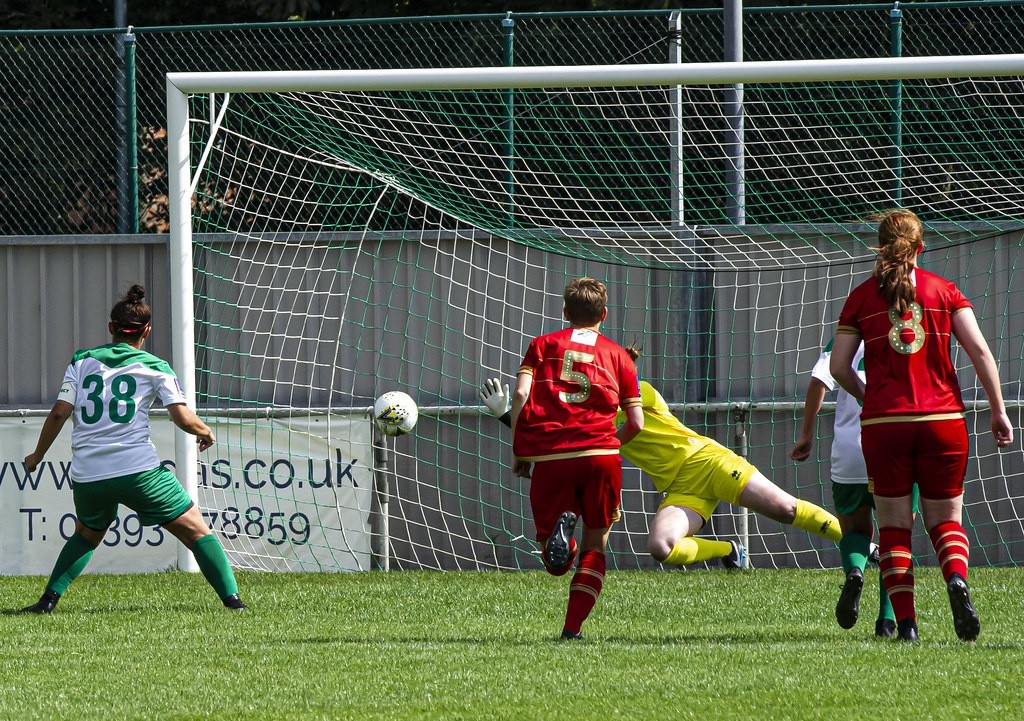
[867,544,880,563]
[20,590,59,615]
[722,539,749,571]
[222,592,247,609]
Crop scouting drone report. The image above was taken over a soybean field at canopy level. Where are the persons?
[790,334,919,638]
[512,277,644,640]
[21,285,252,613]
[480,346,880,570]
[829,210,1013,642]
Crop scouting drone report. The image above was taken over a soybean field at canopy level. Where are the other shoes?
[543,511,578,569]
[898,620,918,643]
[947,576,980,641]
[836,569,863,630]
[875,618,895,637]
[561,628,584,640]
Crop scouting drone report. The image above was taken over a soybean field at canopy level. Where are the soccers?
[371,390,419,437]
